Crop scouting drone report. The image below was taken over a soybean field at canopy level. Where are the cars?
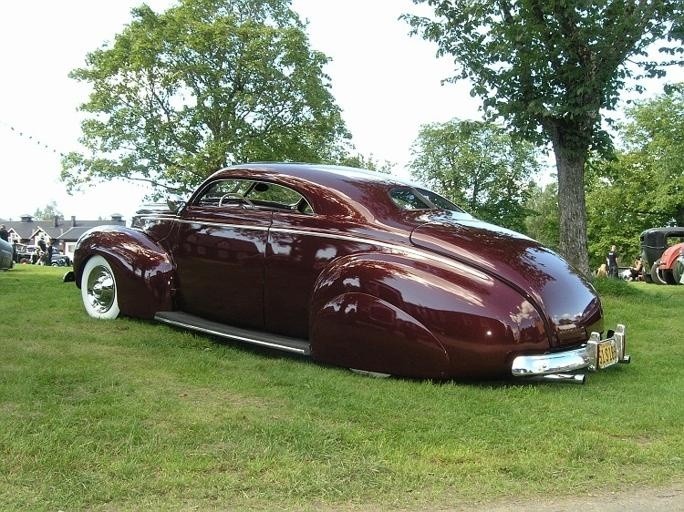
[0,237,69,270]
[62,161,630,384]
[639,227,684,285]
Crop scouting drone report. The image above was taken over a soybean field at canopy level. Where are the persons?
[46,242,52,264]
[629,255,643,279]
[36,234,47,266]
[0,223,8,241]
[607,244,619,279]
[12,240,22,252]
